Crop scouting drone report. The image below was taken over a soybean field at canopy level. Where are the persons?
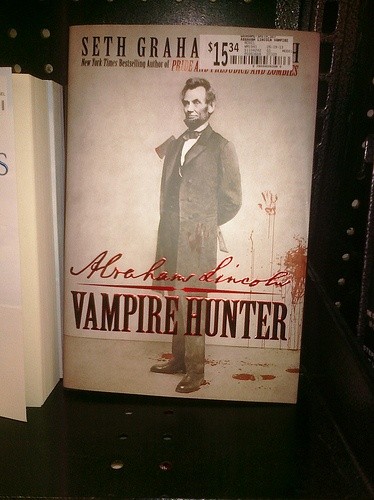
[150,73,242,394]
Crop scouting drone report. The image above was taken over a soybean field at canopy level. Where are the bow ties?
[183,130,202,140]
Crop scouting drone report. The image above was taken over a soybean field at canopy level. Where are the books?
[62,22,321,407]
[0,61,63,422]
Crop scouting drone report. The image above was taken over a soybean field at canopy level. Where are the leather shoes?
[176,373,205,392]
[151,360,186,375]
